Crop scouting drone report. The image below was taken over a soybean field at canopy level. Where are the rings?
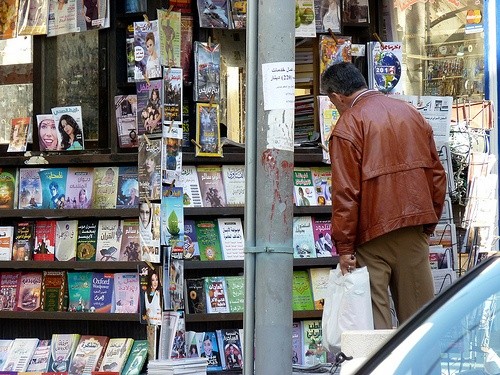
[348,265,350,270]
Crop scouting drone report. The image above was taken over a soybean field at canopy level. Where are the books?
[0,0,405,375]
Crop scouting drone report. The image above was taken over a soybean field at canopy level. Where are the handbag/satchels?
[322,263,375,352]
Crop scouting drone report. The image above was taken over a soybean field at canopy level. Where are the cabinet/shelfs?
[415,25,486,108]
[181,151,341,375]
[0,153,157,375]
[238,33,323,145]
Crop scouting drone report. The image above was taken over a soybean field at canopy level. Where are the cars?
[353,250,500,375]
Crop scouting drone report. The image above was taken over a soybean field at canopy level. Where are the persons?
[322,62,448,331]
[297,187,309,206]
[82,0,100,31]
[11,124,21,145]
[140,150,159,199]
[58,114,83,150]
[190,345,199,358]
[145,32,161,78]
[52,0,70,35]
[127,188,138,206]
[38,115,58,151]
[201,336,220,366]
[144,271,162,324]
[102,362,118,371]
[48,181,60,209]
[227,345,238,365]
[142,88,161,133]
[139,199,153,241]
